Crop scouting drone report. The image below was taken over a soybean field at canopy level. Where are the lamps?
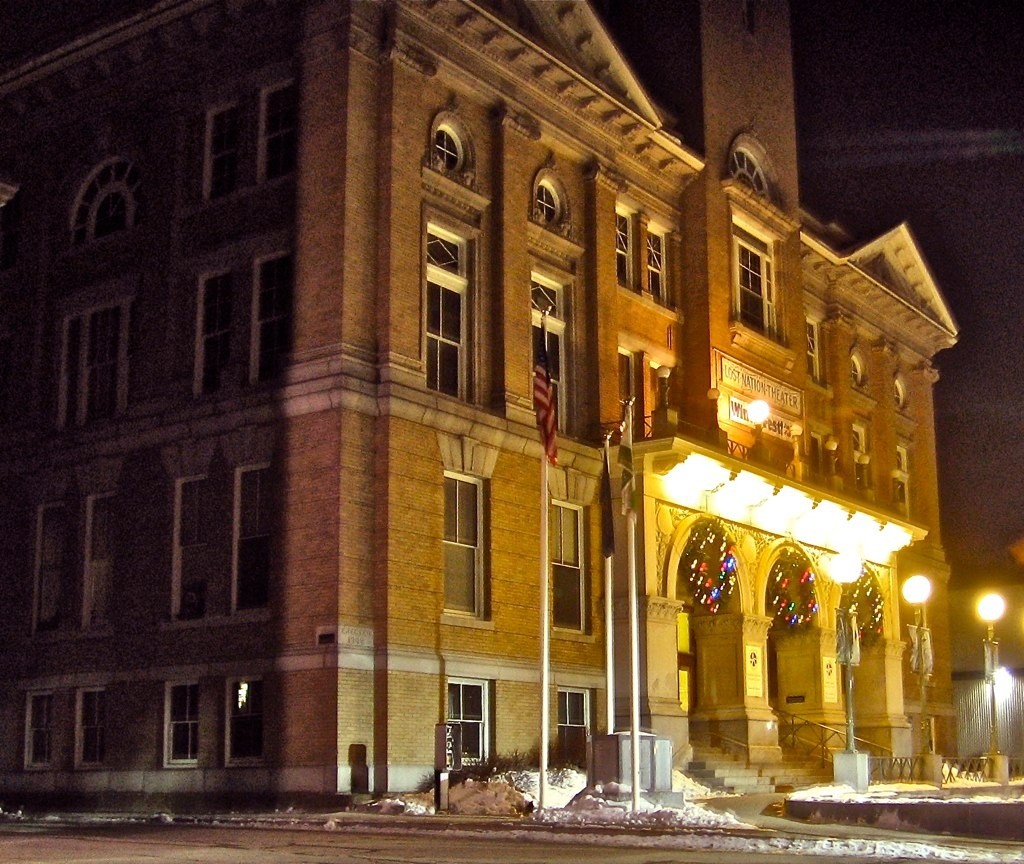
[890,469,899,503]
[748,401,769,444]
[656,366,671,408]
[859,454,871,488]
[790,424,804,461]
[707,389,723,431]
[825,440,837,476]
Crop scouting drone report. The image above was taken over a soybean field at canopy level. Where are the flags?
[534,325,558,466]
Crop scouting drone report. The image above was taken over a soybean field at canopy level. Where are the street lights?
[976,593,1010,782]
[826,552,869,793]
[902,574,942,787]
